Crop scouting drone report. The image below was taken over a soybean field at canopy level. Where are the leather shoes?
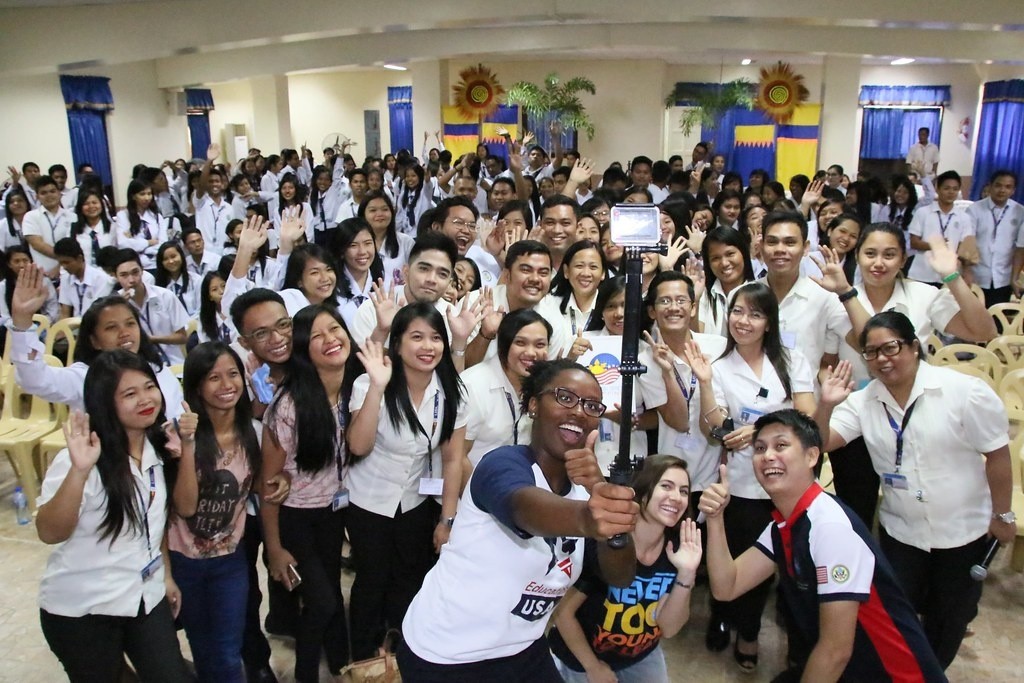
[706,615,731,650]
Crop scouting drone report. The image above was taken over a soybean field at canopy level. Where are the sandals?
[735,631,759,672]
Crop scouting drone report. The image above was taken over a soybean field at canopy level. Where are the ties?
[89,231,100,265]
[250,271,256,284]
[142,220,155,259]
[222,322,232,347]
[173,283,189,316]
[892,215,903,229]
[319,199,326,222]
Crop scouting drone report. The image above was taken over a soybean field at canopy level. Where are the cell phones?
[288,563,301,588]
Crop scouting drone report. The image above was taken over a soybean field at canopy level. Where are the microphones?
[970,539,1000,581]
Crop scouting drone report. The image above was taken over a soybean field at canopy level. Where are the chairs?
[921,282,1024,571]
[0,314,82,513]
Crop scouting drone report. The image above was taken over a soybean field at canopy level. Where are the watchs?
[991,510,1017,524]
[440,512,457,527]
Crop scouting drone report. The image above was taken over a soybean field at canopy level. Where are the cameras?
[710,425,729,440]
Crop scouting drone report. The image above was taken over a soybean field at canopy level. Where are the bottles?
[12,486,32,525]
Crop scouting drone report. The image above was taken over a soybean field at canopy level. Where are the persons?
[697,408,948,683]
[905,127,939,177]
[395,358,638,683]
[0,119,1024,683]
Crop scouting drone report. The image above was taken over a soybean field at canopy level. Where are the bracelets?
[630,413,639,431]
[674,578,695,589]
[450,343,468,355]
[940,268,963,282]
[9,324,26,332]
[838,287,859,302]
[479,325,496,340]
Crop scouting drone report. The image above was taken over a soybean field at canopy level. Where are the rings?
[741,435,744,441]
[75,431,81,436]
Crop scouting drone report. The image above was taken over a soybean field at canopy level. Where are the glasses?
[445,217,480,234]
[535,386,607,418]
[729,307,766,320]
[554,178,566,185]
[654,294,691,306]
[242,317,293,342]
[861,340,908,361]
[592,210,610,217]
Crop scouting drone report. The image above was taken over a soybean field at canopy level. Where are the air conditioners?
[225,123,249,174]
